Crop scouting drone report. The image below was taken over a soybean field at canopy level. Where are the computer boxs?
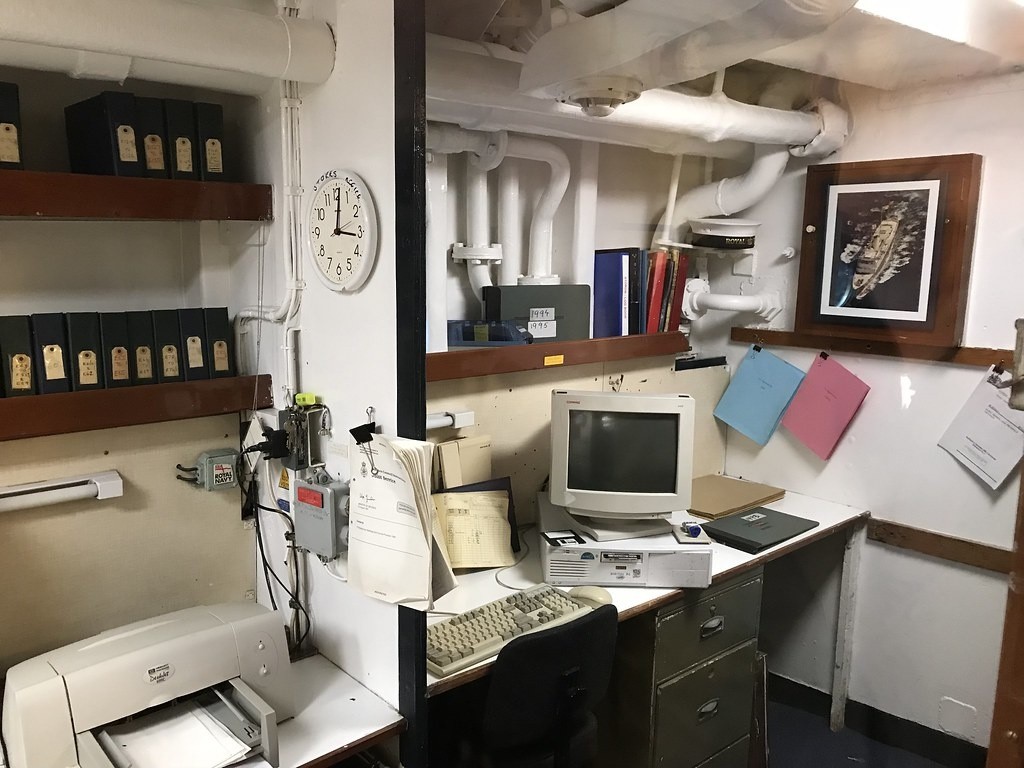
[536,491,713,588]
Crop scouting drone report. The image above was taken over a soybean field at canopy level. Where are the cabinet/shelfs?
[598,560,764,768]
[0,166,272,436]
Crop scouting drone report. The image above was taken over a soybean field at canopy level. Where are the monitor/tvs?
[548,389,696,541]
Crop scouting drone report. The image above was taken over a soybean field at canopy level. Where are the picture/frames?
[793,152,984,349]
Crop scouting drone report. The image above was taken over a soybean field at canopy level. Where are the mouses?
[568,586,613,609]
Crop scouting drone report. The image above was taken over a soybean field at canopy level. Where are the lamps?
[0,469,124,514]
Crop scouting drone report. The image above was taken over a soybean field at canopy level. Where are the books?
[593,247,690,338]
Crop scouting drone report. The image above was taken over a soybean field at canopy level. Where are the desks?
[421,489,873,733]
[272,653,407,768]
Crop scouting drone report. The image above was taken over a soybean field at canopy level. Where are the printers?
[1,600,299,768]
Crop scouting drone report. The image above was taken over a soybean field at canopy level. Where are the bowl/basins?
[687,218,761,249]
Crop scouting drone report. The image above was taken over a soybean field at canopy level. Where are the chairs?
[444,602,618,768]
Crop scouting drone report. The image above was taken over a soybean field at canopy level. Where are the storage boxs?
[100,311,132,390]
[66,312,106,391]
[33,311,71,394]
[0,82,24,168]
[134,95,171,179]
[176,308,209,381]
[193,101,230,181]
[165,98,201,180]
[203,307,236,378]
[64,90,145,176]
[0,314,36,396]
[124,311,158,385]
[151,309,185,384]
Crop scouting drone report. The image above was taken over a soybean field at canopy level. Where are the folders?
[698,506,820,555]
[779,350,871,461]
[594,247,629,337]
[713,343,806,446]
[686,473,786,521]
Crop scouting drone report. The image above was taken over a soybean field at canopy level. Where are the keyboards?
[425,582,592,677]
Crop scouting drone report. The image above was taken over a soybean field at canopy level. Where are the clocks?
[305,167,379,294]
